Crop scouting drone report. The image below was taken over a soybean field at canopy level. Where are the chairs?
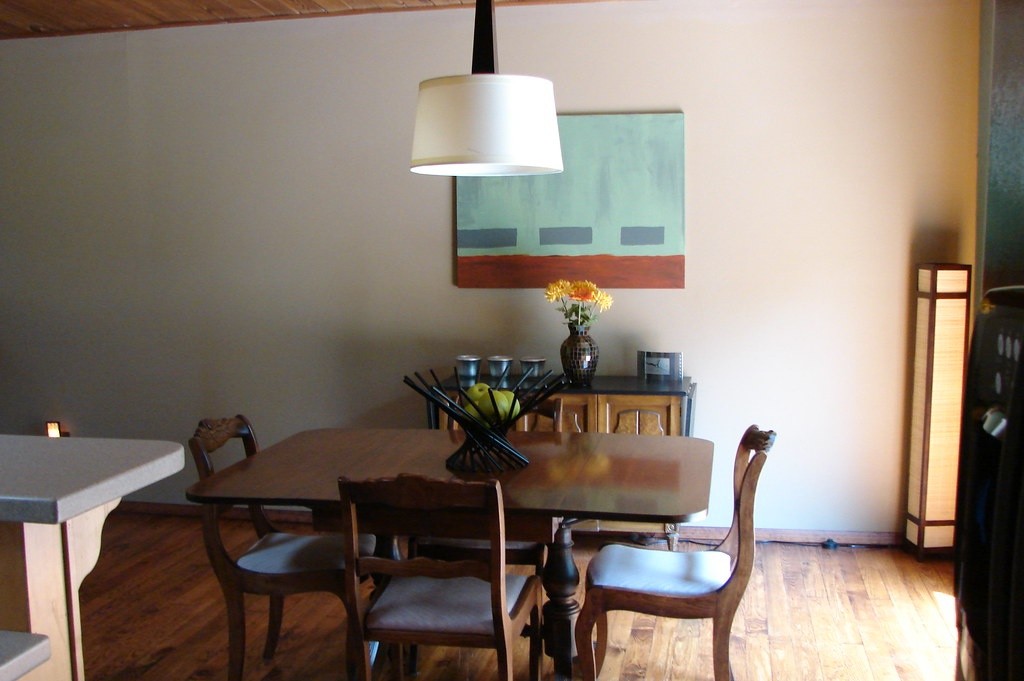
[410,394,564,669]
[337,474,545,681]
[575,424,778,681]
[189,415,387,681]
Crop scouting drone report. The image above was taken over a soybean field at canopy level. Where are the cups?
[487,355,513,379]
[520,359,545,377]
[456,355,482,377]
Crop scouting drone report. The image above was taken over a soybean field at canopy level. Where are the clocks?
[636,351,684,383]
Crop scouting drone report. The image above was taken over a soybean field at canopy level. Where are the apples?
[462,383,520,428]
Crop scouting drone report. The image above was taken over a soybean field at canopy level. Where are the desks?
[185,427,716,681]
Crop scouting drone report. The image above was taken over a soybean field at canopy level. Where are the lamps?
[903,263,973,563]
[408,1,563,179]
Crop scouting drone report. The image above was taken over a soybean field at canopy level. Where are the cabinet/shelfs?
[428,374,697,552]
[0,432,184,681]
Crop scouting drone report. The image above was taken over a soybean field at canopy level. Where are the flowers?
[544,279,614,325]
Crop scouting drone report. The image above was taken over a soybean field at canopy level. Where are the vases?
[560,324,600,387]
